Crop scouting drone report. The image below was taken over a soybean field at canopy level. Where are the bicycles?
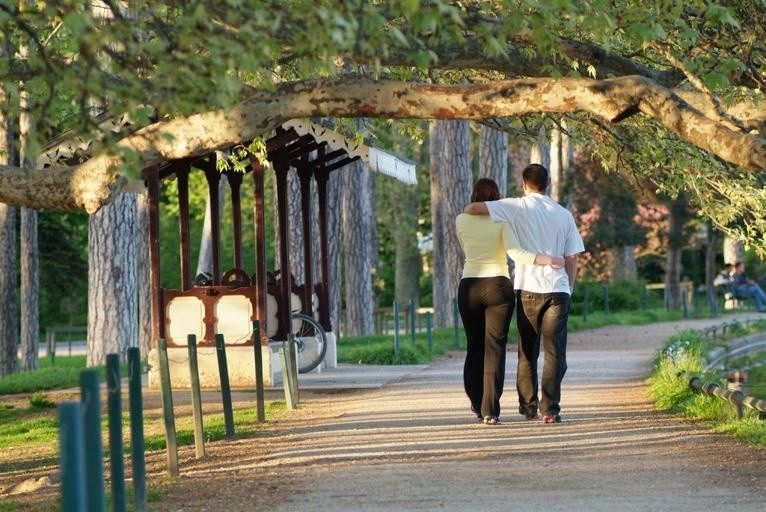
[291,308,329,375]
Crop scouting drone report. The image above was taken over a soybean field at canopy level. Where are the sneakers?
[527,412,562,424]
[471,404,501,425]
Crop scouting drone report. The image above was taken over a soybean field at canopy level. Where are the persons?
[713,262,766,312]
[464,163,586,423]
[454,179,566,425]
